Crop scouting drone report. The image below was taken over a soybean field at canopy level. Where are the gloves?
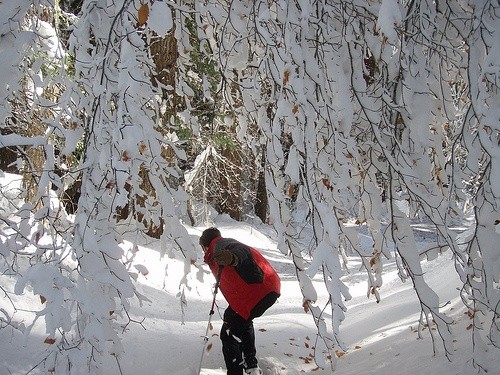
[212,248,232,265]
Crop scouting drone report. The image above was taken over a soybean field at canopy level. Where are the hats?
[199,227,221,248]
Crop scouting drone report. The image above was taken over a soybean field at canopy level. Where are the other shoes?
[245,365,264,375]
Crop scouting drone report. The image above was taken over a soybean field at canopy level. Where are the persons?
[199,227,282,375]
